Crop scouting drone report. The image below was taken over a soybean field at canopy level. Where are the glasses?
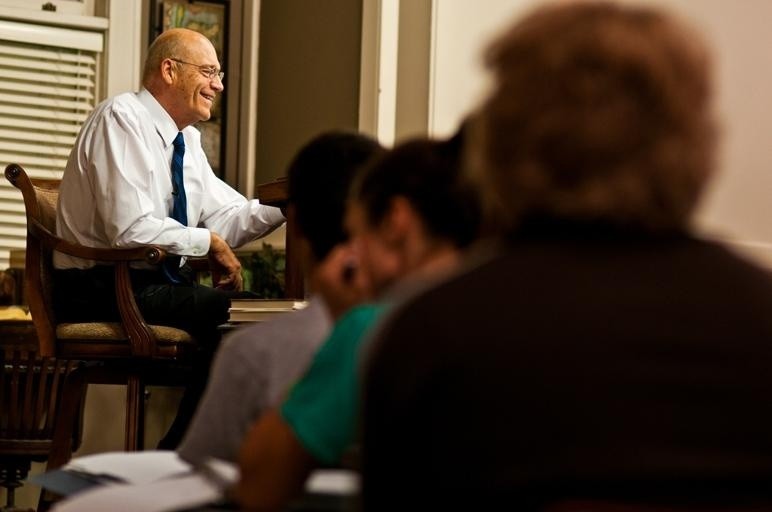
[172,58,225,81]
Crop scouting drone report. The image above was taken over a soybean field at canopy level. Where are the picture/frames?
[149,0,228,181]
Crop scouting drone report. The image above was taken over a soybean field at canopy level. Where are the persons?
[173,130,393,467]
[351,0,771,509]
[218,132,499,510]
[50,28,288,455]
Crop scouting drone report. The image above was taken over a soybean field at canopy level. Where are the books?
[227,307,284,322]
[229,296,310,311]
[309,468,358,495]
[53,450,239,511]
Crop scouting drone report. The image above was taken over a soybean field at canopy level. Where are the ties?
[163,132,188,284]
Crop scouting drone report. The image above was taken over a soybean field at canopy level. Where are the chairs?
[4,165,218,455]
